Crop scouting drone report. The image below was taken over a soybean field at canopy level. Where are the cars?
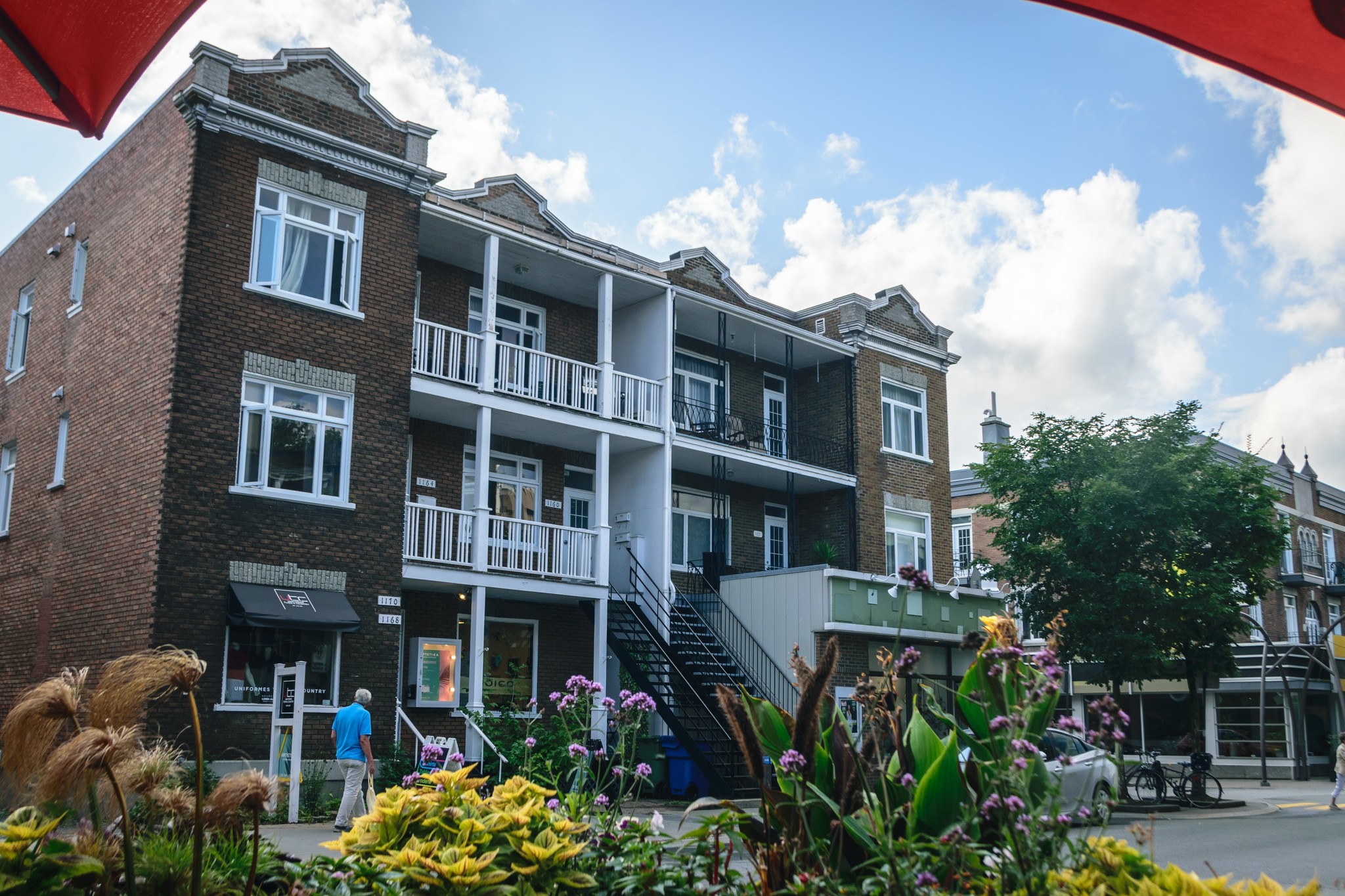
[940,726,1121,828]
[1219,729,1255,758]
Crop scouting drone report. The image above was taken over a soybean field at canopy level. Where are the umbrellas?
[0,0,1345,142]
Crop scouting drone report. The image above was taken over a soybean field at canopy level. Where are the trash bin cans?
[660,735,711,797]
[634,736,668,797]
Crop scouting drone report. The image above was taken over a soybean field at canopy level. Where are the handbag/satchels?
[366,770,377,814]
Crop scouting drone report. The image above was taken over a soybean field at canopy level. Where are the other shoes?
[1329,804,1340,810]
[333,825,353,832]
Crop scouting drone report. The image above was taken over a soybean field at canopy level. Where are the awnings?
[225,582,361,632]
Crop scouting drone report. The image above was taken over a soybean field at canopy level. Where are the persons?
[1329,732,1345,810]
[224,641,286,704]
[1048,716,1056,727]
[330,688,375,833]
[844,704,854,733]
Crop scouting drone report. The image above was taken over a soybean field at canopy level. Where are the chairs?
[684,400,768,455]
[702,552,738,593]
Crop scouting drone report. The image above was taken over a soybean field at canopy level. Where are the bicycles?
[1125,750,1223,809]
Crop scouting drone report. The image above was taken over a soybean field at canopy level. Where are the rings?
[370,771,373,772]
